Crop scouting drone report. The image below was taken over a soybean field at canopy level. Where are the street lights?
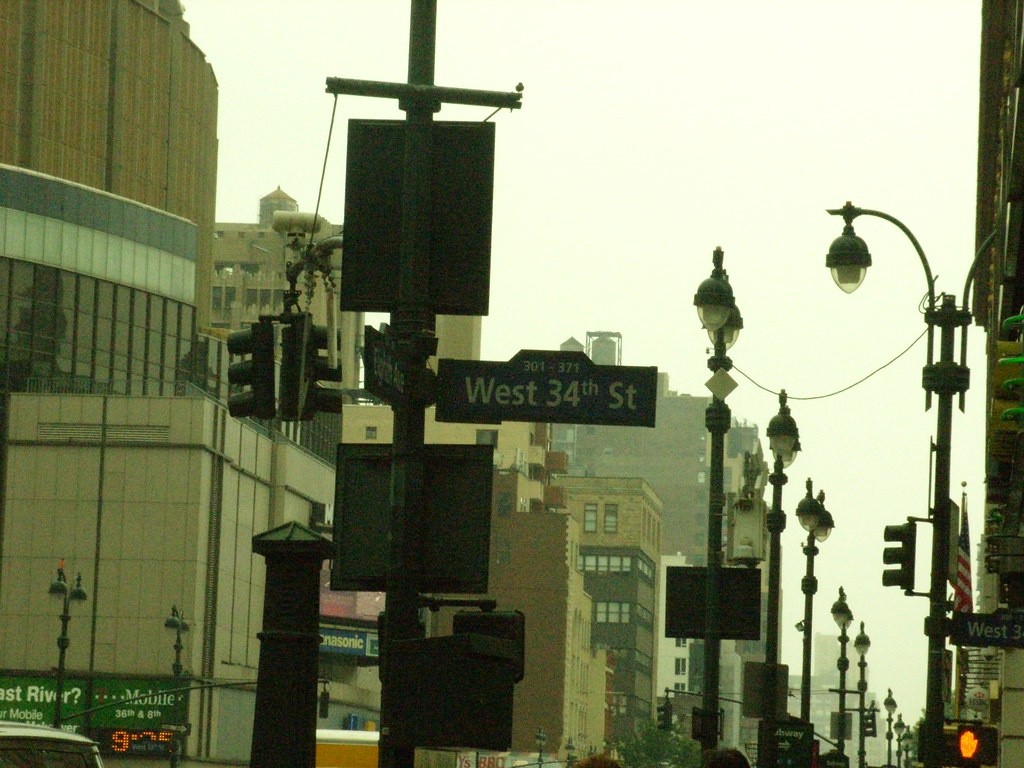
[536,729,546,768]
[794,477,837,721]
[47,568,88,729]
[832,587,917,768]
[825,196,1024,768]
[165,604,190,768]
[765,388,806,664]
[692,247,740,768]
[565,738,579,768]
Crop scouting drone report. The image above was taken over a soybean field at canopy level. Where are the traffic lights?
[656,706,670,731]
[956,724,999,768]
[310,324,344,422]
[882,524,908,588]
[227,325,261,419]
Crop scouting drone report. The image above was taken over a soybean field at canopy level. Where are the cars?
[0,721,107,768]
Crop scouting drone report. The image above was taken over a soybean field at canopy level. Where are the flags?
[954,496,973,612]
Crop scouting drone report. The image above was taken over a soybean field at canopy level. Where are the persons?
[699,748,751,768]
[572,755,620,768]
